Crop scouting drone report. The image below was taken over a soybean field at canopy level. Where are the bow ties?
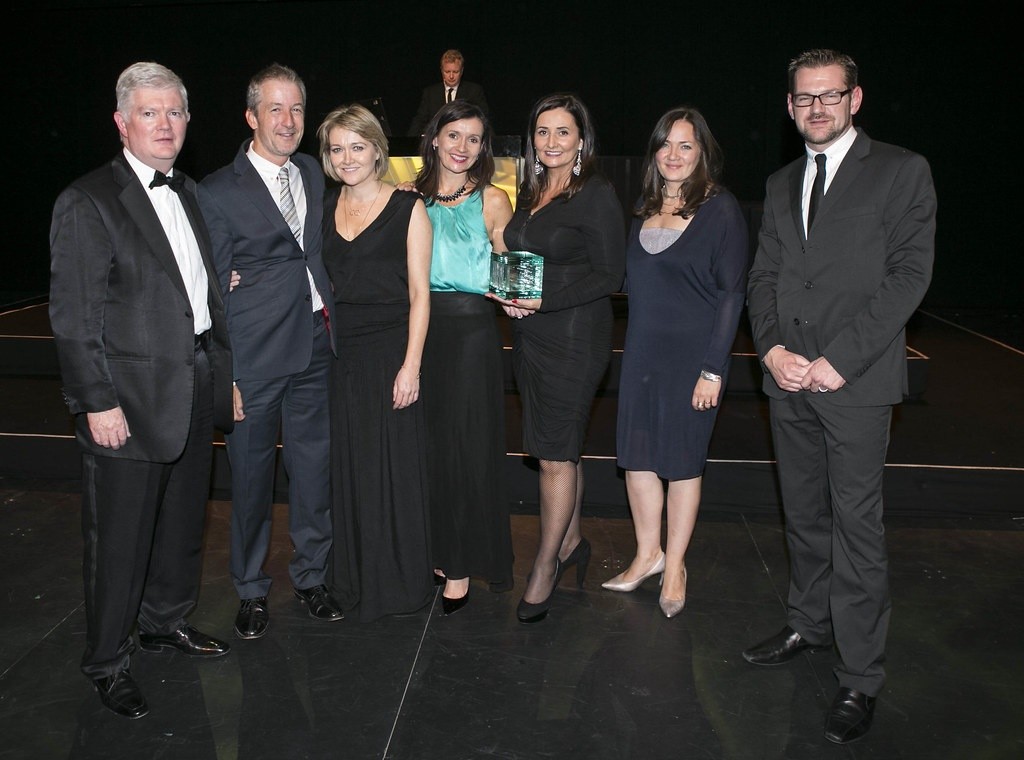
[148,170,187,192]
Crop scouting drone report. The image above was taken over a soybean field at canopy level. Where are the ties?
[277,166,303,243]
[806,153,827,236]
[448,89,453,102]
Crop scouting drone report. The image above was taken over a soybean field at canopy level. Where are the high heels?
[602,553,667,592]
[659,568,688,619]
[516,557,563,623]
[528,537,590,588]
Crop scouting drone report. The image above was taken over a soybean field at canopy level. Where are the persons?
[416,103,536,614]
[198,69,418,640]
[226,107,434,624]
[485,97,626,623]
[417,50,490,138]
[49,67,236,718]
[742,46,937,744]
[602,107,748,618]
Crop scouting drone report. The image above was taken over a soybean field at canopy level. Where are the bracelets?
[701,370,721,382]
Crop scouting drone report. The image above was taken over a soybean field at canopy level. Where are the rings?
[698,402,705,407]
[819,386,828,392]
[518,315,522,319]
[705,401,711,404]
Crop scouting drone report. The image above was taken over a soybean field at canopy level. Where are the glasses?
[790,90,850,107]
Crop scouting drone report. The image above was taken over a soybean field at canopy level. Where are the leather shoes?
[824,687,875,745]
[139,625,231,658]
[294,584,345,622]
[91,670,151,719]
[234,597,268,640]
[742,625,833,666]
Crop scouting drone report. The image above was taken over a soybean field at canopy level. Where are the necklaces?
[437,178,471,203]
[662,185,681,199]
[344,180,383,239]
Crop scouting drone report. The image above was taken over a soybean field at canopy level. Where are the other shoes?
[442,577,470,616]
[434,571,445,587]
